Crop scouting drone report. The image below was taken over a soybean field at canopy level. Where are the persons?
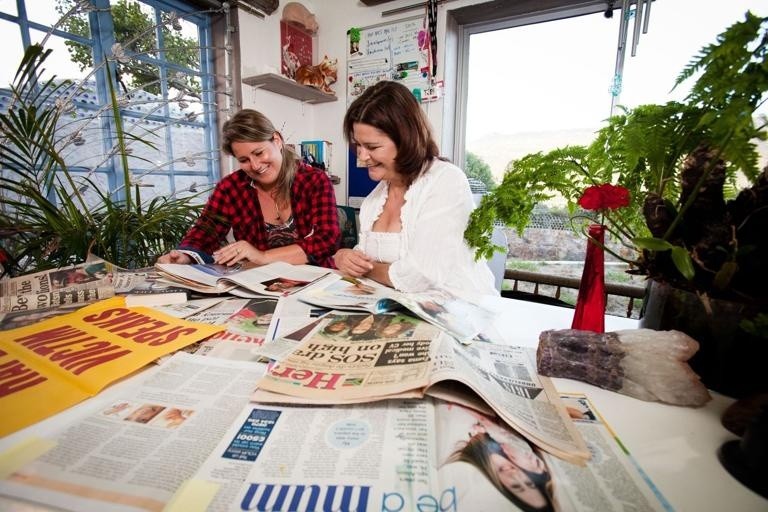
[325,314,420,338]
[333,81,505,314]
[128,408,161,422]
[157,108,343,271]
[417,296,467,323]
[262,278,309,291]
[441,415,562,511]
[65,263,106,283]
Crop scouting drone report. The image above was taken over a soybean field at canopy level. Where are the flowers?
[576,182,630,321]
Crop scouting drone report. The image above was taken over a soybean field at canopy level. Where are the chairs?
[484,226,508,293]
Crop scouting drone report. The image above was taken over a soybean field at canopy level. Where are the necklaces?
[262,187,282,220]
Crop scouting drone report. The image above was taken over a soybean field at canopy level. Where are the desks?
[0,266,768,512]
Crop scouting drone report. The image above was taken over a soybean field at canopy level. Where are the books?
[154,258,331,295]
[297,272,482,345]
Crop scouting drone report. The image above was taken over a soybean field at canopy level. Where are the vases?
[571,224,607,334]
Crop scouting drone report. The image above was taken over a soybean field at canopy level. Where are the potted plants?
[464,12,768,400]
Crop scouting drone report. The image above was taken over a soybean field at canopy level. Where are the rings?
[236,248,239,254]
